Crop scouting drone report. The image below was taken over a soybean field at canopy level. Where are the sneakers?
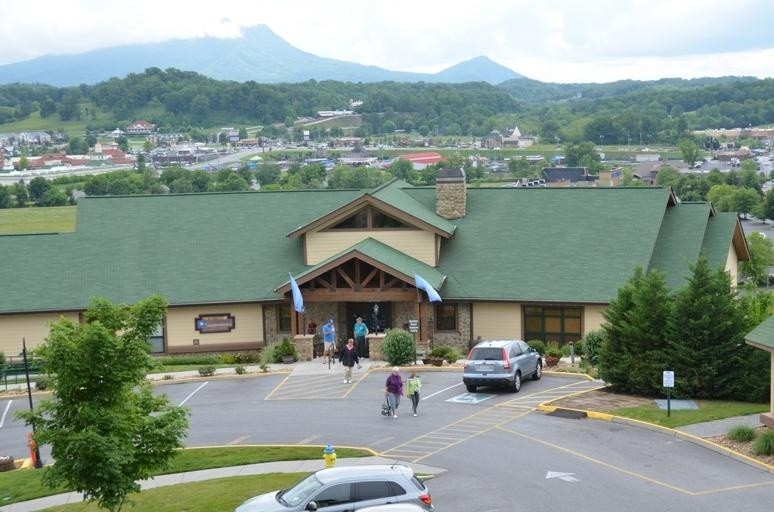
[342,378,353,384]
[392,404,419,420]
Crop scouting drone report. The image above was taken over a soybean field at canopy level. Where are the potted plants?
[280,336,295,363]
[544,347,563,366]
[423,346,457,367]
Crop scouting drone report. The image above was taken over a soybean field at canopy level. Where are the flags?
[415,274,442,304]
[288,272,305,313]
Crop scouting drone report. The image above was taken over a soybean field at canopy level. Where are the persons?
[320,318,339,365]
[405,370,421,417]
[338,338,359,384]
[352,316,368,361]
[384,366,403,419]
[305,319,316,334]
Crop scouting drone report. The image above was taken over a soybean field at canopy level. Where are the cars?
[240,465,435,512]
[463,338,542,393]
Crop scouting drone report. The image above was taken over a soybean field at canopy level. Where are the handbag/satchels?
[381,397,391,416]
